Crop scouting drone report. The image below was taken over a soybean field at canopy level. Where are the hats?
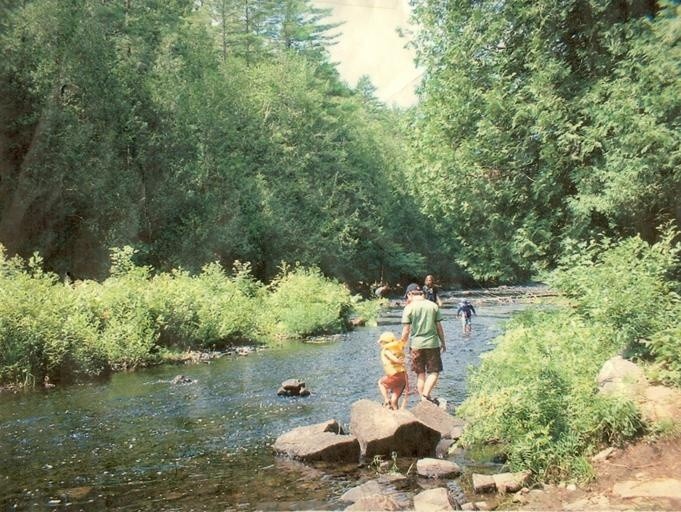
[376,330,398,343]
[404,283,421,299]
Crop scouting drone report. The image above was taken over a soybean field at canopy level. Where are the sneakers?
[382,399,392,409]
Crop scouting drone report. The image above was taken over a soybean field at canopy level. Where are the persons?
[454,298,477,334]
[398,282,447,405]
[420,273,443,310]
[376,331,408,411]
